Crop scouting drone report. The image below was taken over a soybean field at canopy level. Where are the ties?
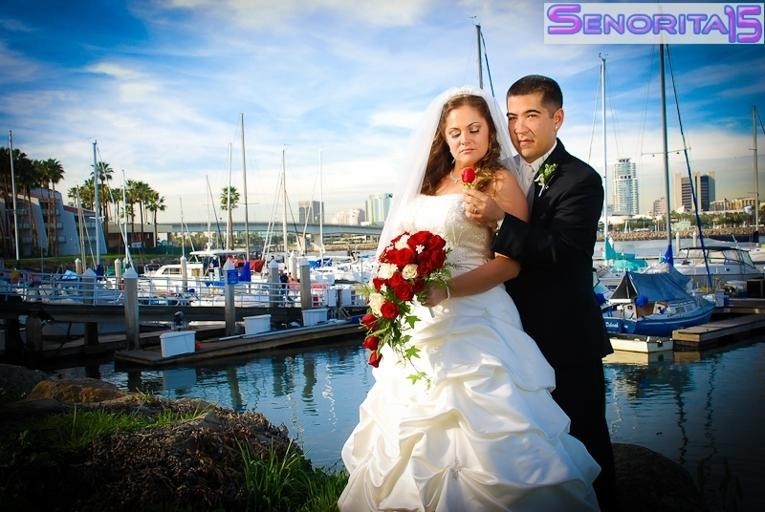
[520,164,533,187]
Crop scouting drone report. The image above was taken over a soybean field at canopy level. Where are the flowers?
[353,231,457,391]
[461,168,497,192]
[534,163,559,197]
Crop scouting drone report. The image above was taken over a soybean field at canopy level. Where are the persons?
[267,255,278,281]
[369,81,536,511]
[456,73,624,510]
[279,269,296,305]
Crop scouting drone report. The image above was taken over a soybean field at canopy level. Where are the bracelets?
[442,279,451,301]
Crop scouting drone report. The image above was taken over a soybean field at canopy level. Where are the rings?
[472,207,479,214]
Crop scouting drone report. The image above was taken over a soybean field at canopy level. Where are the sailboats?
[0,25,765,341]
